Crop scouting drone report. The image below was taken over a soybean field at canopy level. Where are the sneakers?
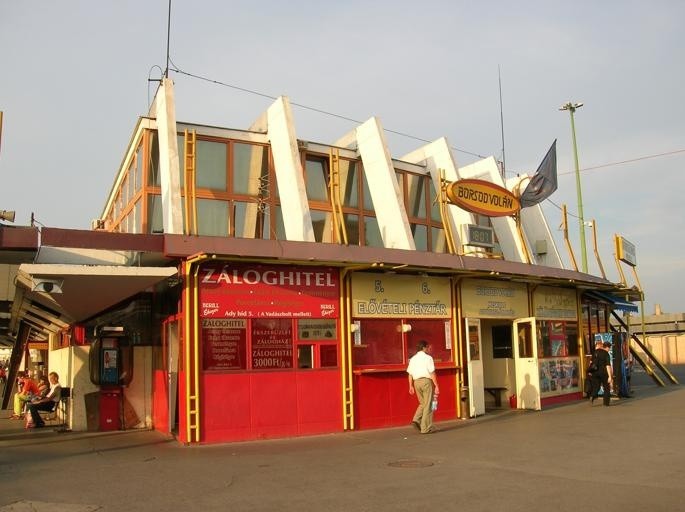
[589,394,595,406]
[411,421,421,433]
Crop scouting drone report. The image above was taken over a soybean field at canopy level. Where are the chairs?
[38,397,65,426]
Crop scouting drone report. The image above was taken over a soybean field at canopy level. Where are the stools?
[482,387,507,406]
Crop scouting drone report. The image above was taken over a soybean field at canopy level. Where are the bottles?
[622,333,631,382]
[431,397,438,411]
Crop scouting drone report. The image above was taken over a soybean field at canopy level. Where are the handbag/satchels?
[587,358,599,373]
[583,375,594,393]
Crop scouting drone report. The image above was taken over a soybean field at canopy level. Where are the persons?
[405,339,440,434]
[0,359,50,420]
[589,340,614,408]
[105,351,111,367]
[26,371,62,428]
